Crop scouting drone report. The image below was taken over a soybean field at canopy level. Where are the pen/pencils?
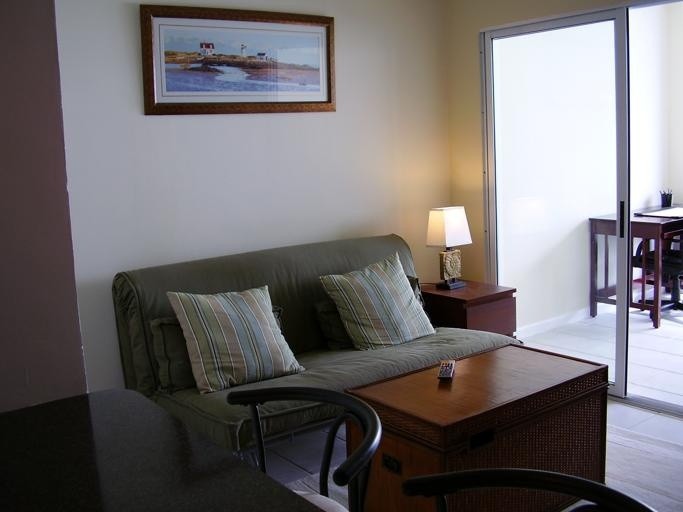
[659,188,673,195]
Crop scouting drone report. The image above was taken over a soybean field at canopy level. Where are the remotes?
[437,359,455,380]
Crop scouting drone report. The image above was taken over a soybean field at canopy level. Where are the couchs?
[109,234,523,470]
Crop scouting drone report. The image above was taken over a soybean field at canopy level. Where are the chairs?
[630,237,683,315]
[221,384,381,512]
[401,465,656,512]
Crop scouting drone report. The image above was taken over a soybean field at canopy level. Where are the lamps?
[426,204,473,291]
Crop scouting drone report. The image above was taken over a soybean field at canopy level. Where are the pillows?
[167,284,304,395]
[320,249,435,351]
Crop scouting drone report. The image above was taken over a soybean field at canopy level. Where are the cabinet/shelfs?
[420,278,516,339]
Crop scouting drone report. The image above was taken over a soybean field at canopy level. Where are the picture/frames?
[140,4,337,117]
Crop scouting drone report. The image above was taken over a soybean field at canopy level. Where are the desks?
[588,206,682,329]
[0,388,322,511]
